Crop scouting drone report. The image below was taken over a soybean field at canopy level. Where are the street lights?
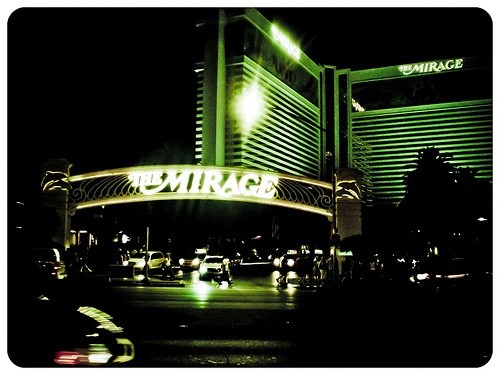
[326,149,338,274]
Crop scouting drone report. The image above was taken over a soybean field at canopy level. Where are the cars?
[129,251,166,270]
[198,255,225,280]
[27,248,63,282]
[7,301,136,367]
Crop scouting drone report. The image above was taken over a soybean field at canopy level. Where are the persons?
[157,253,176,280]
[71,248,131,273]
[218,255,234,285]
[275,251,420,288]
